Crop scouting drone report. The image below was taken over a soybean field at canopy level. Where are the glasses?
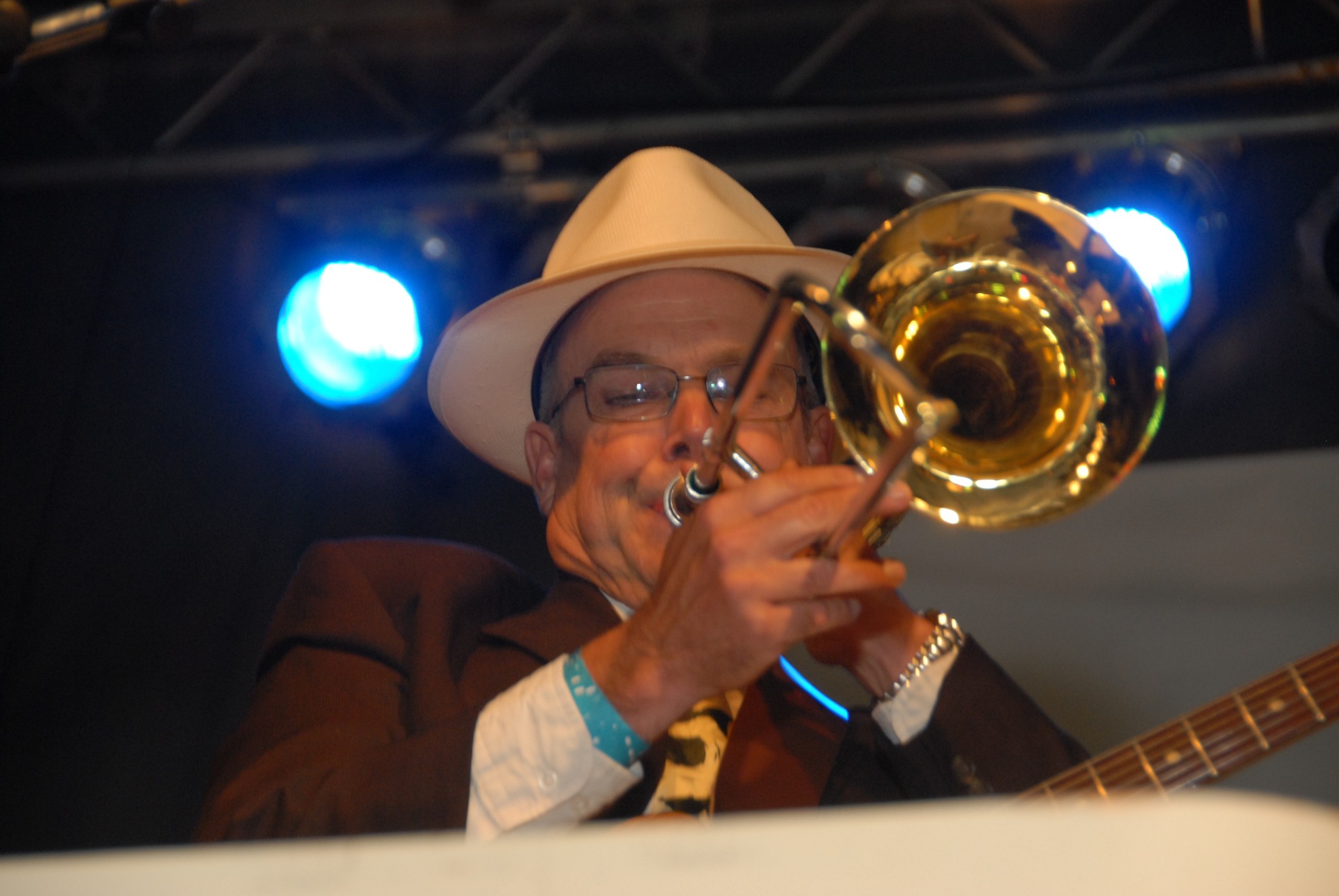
[546,364,820,421]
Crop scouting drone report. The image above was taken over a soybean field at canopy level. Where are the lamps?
[790,159,950,251]
[1074,151,1234,372]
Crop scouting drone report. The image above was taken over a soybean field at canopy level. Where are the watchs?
[876,608,966,701]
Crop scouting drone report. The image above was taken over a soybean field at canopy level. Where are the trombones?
[662,186,1171,559]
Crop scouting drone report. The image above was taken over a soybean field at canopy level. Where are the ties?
[651,688,743,821]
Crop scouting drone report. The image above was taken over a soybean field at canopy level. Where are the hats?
[427,146,853,487]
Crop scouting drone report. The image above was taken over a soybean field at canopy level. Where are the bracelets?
[564,652,651,767]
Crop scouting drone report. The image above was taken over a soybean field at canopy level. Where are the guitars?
[1012,645,1338,801]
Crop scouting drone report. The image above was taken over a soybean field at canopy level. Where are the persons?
[194,145,1091,840]
